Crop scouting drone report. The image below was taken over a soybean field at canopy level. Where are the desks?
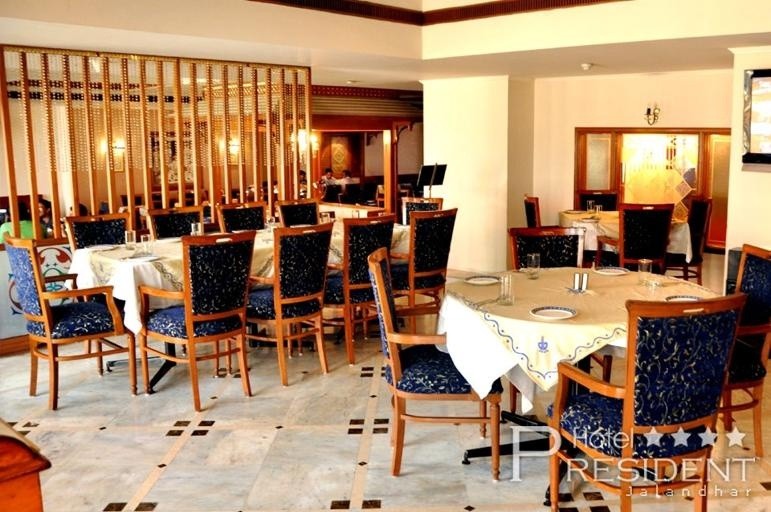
[0,437,53,512]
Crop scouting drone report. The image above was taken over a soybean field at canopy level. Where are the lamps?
[644,104,661,126]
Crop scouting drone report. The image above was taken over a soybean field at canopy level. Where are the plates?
[580,219,599,223]
[125,255,158,261]
[463,275,499,286]
[528,306,577,321]
[566,210,582,213]
[595,267,629,276]
[665,294,702,302]
[84,244,118,250]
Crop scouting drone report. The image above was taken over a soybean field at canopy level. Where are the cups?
[596,205,602,218]
[141,234,151,252]
[587,201,594,212]
[498,274,516,306]
[352,210,359,218]
[321,213,329,223]
[526,254,540,280]
[125,231,136,249]
[267,217,275,232]
[647,277,662,298]
[191,222,202,235]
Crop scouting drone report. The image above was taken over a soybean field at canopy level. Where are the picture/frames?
[742,65,771,166]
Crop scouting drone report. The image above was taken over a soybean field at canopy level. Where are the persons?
[299,170,307,192]
[59,202,88,238]
[38,198,52,228]
[0,200,47,243]
[335,170,355,203]
[321,168,336,186]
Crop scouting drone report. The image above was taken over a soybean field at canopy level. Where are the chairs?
[322,184,341,203]
[386,208,459,333]
[275,198,319,225]
[339,182,362,204]
[216,202,266,233]
[368,246,502,471]
[68,211,131,251]
[508,224,587,268]
[2,230,139,411]
[711,244,771,459]
[401,195,442,225]
[139,227,258,413]
[577,188,622,211]
[144,206,204,240]
[523,194,541,228]
[325,212,398,364]
[595,202,675,272]
[244,221,336,386]
[550,291,747,512]
[664,194,714,285]
